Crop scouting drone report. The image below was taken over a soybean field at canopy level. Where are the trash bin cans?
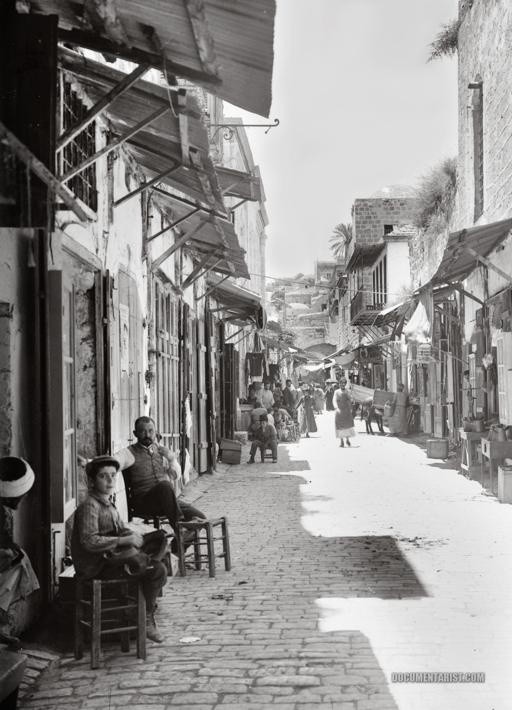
[497,465,512,504]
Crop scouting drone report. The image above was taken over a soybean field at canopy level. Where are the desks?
[457,427,488,480]
[479,435,512,492]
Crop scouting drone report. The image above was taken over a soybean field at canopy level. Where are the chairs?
[121,468,173,538]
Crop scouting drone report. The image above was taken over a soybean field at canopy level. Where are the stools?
[259,447,278,463]
[176,515,232,578]
[58,564,140,641]
[286,422,299,441]
[70,573,147,669]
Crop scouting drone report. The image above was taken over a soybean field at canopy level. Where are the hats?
[257,415,268,420]
[85,456,119,477]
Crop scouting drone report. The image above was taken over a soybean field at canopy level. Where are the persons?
[0,457,40,644]
[248,378,409,464]
[77,417,207,558]
[72,455,169,643]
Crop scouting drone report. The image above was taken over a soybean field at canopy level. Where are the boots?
[169,521,195,541]
[171,530,195,554]
[128,601,163,643]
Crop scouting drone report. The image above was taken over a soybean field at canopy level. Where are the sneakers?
[339,440,350,447]
[247,459,254,463]
[272,458,277,463]
